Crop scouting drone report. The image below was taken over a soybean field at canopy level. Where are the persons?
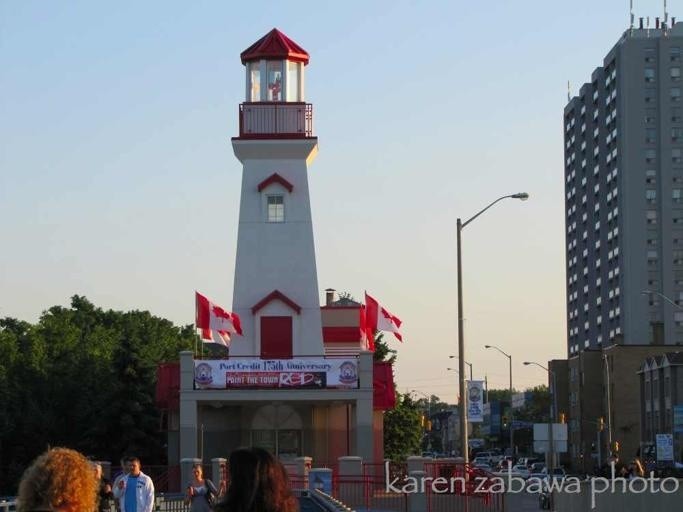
[601,454,644,479]
[94,457,155,512]
[15,447,101,512]
[214,448,300,512]
[184,464,226,512]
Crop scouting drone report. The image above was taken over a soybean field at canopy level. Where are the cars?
[421,450,566,494]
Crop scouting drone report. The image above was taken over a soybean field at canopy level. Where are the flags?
[195,291,244,347]
[360,291,403,352]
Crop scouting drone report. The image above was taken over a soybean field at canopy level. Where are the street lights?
[640,290,683,316]
[485,344,512,420]
[524,361,555,452]
[447,354,472,380]
[456,192,528,467]
